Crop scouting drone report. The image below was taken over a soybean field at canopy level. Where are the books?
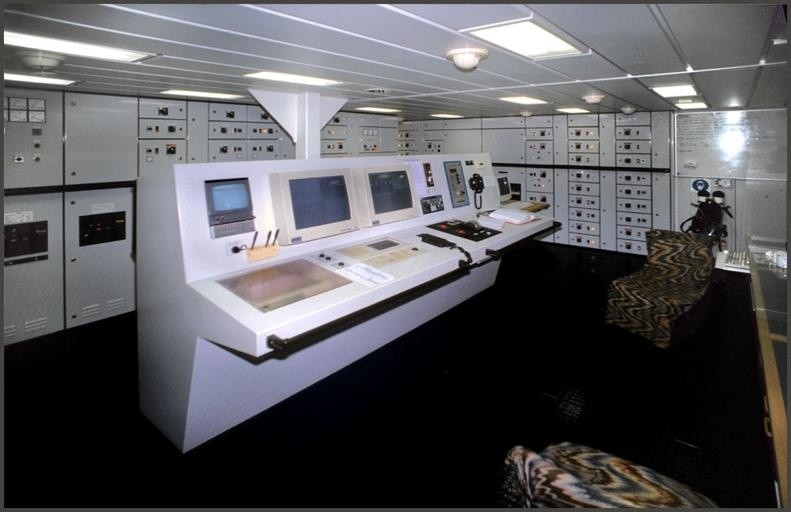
[478,215,506,231]
[490,207,544,225]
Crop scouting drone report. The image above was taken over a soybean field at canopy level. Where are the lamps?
[447,48,487,69]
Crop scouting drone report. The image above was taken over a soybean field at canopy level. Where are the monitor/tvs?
[345,165,424,228]
[270,169,362,247]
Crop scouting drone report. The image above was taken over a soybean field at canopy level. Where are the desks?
[746,244,788,508]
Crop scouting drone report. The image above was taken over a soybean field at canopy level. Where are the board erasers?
[683,161,695,166]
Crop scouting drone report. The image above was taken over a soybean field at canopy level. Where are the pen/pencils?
[265,231,271,247]
[251,231,258,249]
[272,229,280,245]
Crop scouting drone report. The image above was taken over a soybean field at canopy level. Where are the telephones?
[470,173,484,193]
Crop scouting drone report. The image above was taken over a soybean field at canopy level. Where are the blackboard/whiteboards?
[674,108,788,182]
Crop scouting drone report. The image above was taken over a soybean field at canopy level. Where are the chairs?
[607,229,713,349]
